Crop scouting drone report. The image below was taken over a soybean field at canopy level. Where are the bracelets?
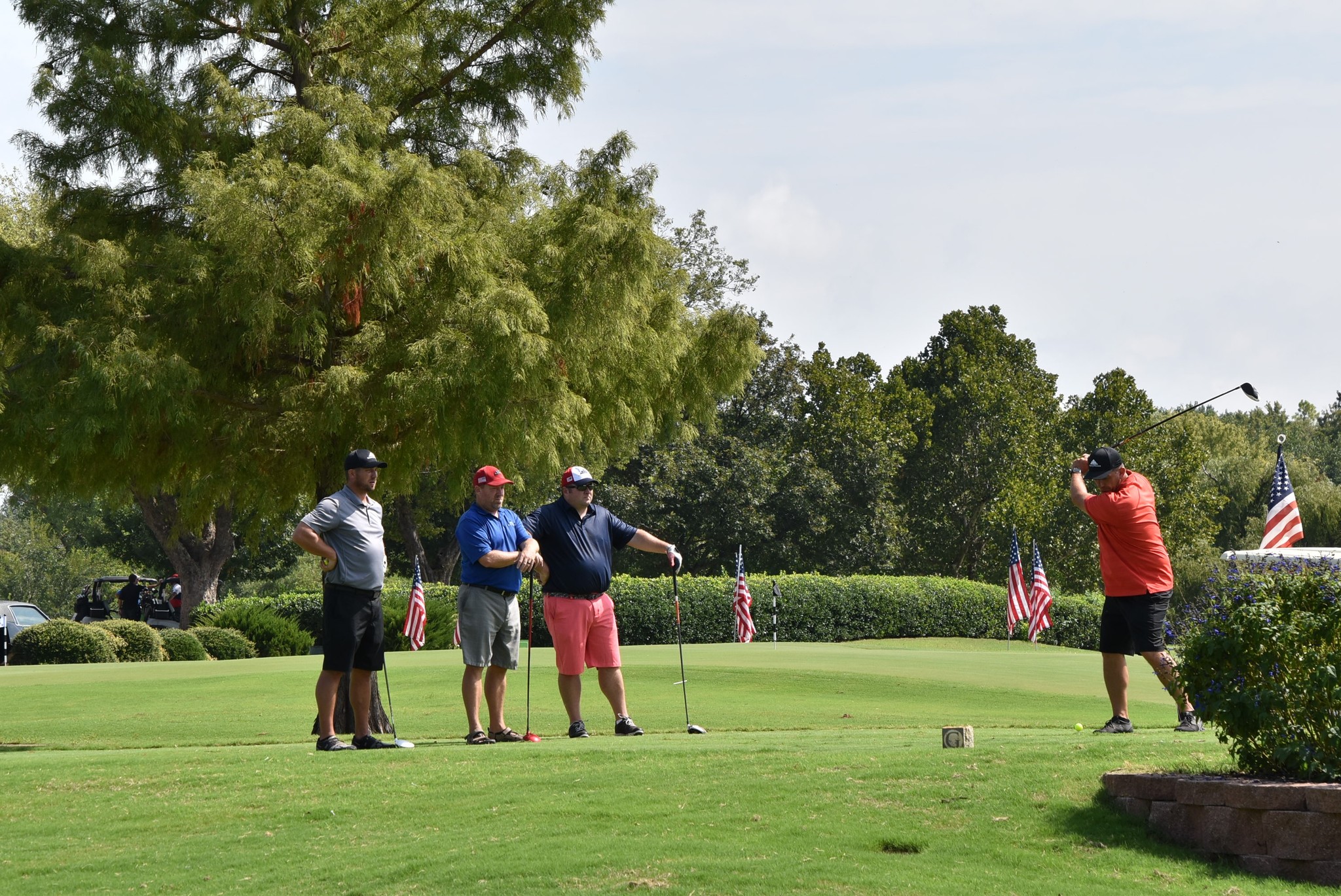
[118,608,122,609]
[665,544,672,555]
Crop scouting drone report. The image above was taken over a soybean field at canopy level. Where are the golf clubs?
[672,557,707,734]
[523,563,541,742]
[1111,382,1261,448]
[140,587,158,601]
[82,584,91,597]
[383,650,416,748]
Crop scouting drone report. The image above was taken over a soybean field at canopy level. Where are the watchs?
[1070,467,1082,477]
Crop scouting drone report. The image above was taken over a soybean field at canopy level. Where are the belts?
[323,584,382,599]
[543,592,604,600]
[461,582,515,597]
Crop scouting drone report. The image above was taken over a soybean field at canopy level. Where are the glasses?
[566,484,595,491]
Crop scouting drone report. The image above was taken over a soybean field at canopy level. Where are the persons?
[456,465,544,745]
[1070,445,1204,734]
[518,466,683,738]
[167,574,182,600]
[294,448,400,750]
[116,574,162,622]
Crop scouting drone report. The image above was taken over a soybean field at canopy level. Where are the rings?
[527,562,531,564]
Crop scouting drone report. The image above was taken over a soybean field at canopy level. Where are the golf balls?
[1075,723,1083,730]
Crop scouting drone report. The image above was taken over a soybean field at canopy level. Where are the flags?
[403,553,426,651]
[1259,445,1304,549]
[732,551,758,643]
[1007,524,1032,636]
[1028,540,1053,643]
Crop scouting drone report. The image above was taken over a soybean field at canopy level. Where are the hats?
[473,466,514,486]
[561,466,599,486]
[1083,446,1123,479]
[344,449,388,470]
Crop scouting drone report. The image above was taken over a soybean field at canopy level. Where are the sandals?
[464,730,496,745]
[487,727,524,742]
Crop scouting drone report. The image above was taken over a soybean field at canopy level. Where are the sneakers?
[615,713,643,736]
[315,735,357,751]
[568,720,590,738]
[1174,710,1205,732]
[1092,716,1134,733]
[351,735,396,750]
